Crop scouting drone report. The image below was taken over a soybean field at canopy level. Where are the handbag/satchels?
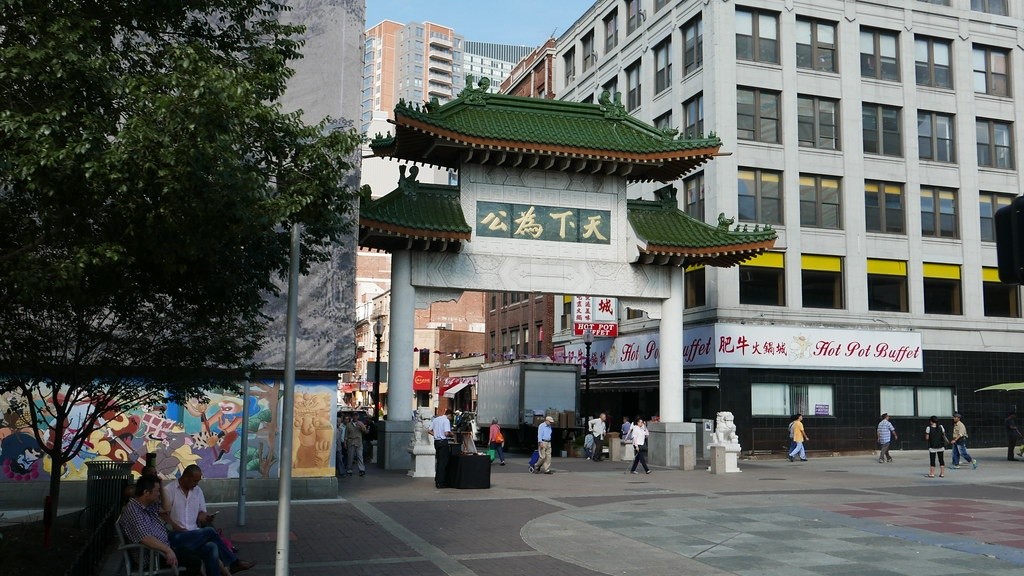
[943,435,950,448]
[638,443,648,451]
[495,433,504,444]
[484,444,496,462]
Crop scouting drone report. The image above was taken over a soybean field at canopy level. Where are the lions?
[712,412,738,444]
[412,407,435,442]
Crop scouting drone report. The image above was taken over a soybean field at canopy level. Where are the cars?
[336,402,387,440]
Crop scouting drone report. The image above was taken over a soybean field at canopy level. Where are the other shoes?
[631,470,638,474]
[972,459,977,469]
[1008,457,1018,461]
[229,559,258,574]
[789,455,793,462]
[950,465,959,469]
[800,458,808,462]
[888,457,894,463]
[436,483,446,488]
[646,470,652,473]
[593,457,602,461]
[544,471,553,475]
[535,464,539,473]
[360,471,365,476]
[879,459,887,462]
[586,457,591,460]
[500,462,505,466]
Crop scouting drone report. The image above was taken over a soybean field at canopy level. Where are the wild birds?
[863,318,890,326]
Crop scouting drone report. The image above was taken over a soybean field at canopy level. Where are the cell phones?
[210,511,220,517]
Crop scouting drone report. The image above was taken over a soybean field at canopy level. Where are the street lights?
[372,314,385,441]
[581,327,593,436]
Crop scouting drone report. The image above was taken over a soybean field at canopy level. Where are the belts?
[542,439,551,443]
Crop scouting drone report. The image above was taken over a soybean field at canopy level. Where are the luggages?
[529,449,540,473]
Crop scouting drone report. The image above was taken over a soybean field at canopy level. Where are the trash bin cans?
[85,460,135,520]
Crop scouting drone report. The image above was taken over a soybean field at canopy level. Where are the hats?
[545,416,554,423]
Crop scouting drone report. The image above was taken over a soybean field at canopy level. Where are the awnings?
[442,380,469,400]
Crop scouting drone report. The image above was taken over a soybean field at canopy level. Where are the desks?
[445,454,491,489]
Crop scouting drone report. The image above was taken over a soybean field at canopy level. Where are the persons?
[444,407,472,444]
[924,415,947,478]
[876,412,898,463]
[584,411,611,462]
[427,408,456,489]
[948,412,977,470]
[621,416,633,440]
[1000,415,1020,461]
[631,418,652,474]
[534,416,554,474]
[487,417,506,465]
[336,411,369,478]
[787,413,810,461]
[119,464,258,576]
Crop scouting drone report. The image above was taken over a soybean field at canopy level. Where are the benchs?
[116,512,194,576]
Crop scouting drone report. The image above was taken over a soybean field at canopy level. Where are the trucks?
[470,361,586,453]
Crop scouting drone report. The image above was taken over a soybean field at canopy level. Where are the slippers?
[925,474,935,477]
[939,474,944,478]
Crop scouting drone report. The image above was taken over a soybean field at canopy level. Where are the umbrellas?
[974,382,1024,392]
